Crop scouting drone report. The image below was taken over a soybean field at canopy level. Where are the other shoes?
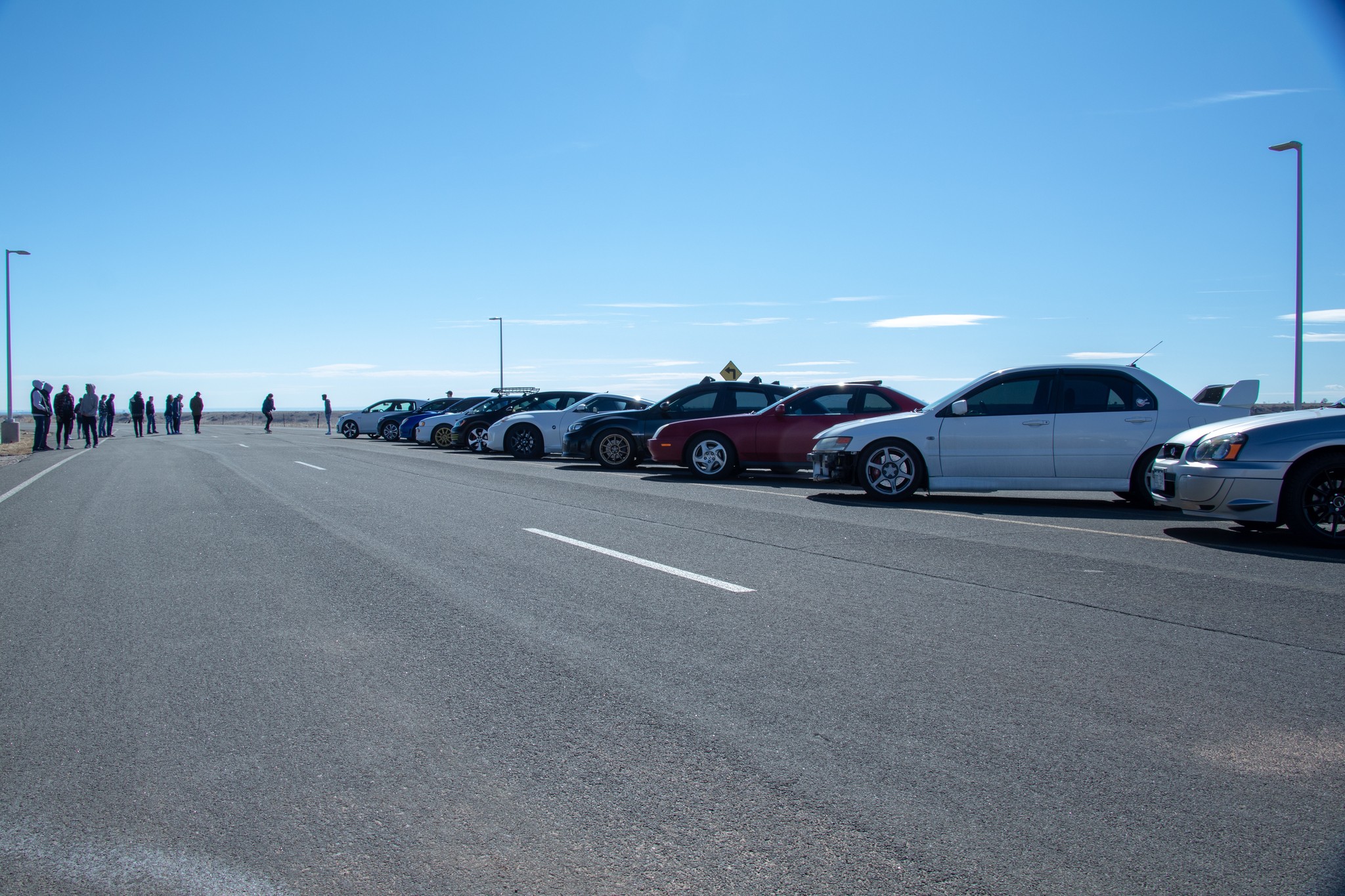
[167,431,182,435]
[140,435,144,437]
[195,430,201,434]
[98,435,105,438]
[136,435,140,438]
[153,431,159,433]
[147,432,153,434]
[265,428,271,431]
[85,443,91,448]
[107,435,112,437]
[83,437,86,439]
[103,435,110,437]
[68,435,75,440]
[93,443,98,447]
[325,432,332,435]
[78,436,80,439]
[110,434,116,437]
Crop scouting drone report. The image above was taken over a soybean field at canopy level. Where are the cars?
[648,380,929,482]
[560,377,833,470]
[377,388,656,461]
[806,341,1260,503]
[336,399,430,439]
[1152,396,1345,551]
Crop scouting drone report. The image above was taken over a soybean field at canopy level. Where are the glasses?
[41,383,43,385]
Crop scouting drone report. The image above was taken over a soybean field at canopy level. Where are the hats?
[176,394,183,397]
[445,391,453,395]
[196,392,202,395]
[42,381,45,383]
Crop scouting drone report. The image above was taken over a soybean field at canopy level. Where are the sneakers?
[32,447,46,452]
[57,445,61,449]
[63,444,74,449]
[41,446,54,450]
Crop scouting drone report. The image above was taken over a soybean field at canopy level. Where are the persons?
[322,394,332,435]
[129,391,145,438]
[146,396,159,434]
[262,393,275,432]
[164,394,178,435]
[190,392,204,434]
[74,398,86,439]
[173,394,183,434]
[31,380,54,453]
[445,391,453,397]
[81,384,98,448]
[106,394,116,437]
[68,413,76,439]
[98,395,109,438]
[53,384,75,449]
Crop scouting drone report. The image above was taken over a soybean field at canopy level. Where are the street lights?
[4,249,31,423]
[489,317,503,395]
[1270,141,1301,410]
